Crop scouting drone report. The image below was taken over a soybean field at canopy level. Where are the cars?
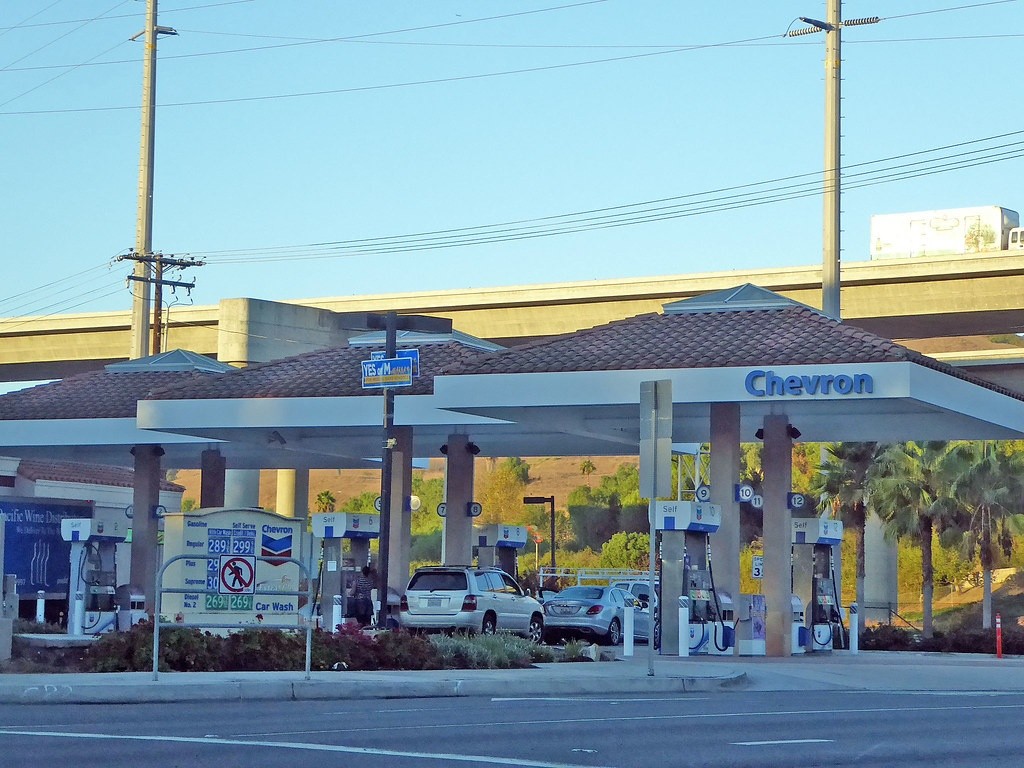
[534,591,557,606]
[543,585,661,650]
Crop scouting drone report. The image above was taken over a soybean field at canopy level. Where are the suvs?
[400,564,543,646]
[610,579,661,614]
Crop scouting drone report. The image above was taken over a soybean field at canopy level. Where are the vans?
[81,592,147,635]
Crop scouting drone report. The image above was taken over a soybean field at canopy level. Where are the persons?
[349,565,375,630]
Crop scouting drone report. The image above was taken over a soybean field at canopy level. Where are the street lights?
[523,495,555,581]
